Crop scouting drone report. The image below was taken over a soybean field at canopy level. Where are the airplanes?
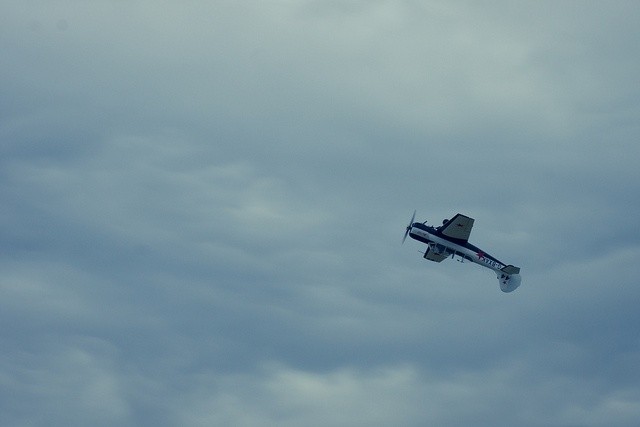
[402,212,522,292]
